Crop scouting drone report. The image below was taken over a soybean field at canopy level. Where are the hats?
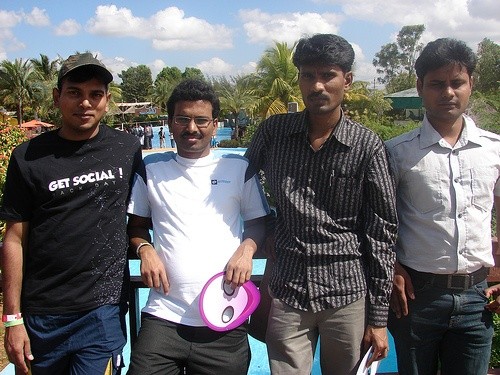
[58,54,113,84]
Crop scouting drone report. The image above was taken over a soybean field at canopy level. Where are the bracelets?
[136,242,154,258]
[2,313,24,327]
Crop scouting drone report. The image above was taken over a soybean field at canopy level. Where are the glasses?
[172,117,214,128]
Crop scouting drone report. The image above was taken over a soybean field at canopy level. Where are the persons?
[125,123,153,149]
[158,127,166,148]
[1,53,142,375]
[126,78,271,375]
[241,34,400,375]
[382,39,500,375]
[170,133,175,148]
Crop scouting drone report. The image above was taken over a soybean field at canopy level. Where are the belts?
[398,267,489,290]
